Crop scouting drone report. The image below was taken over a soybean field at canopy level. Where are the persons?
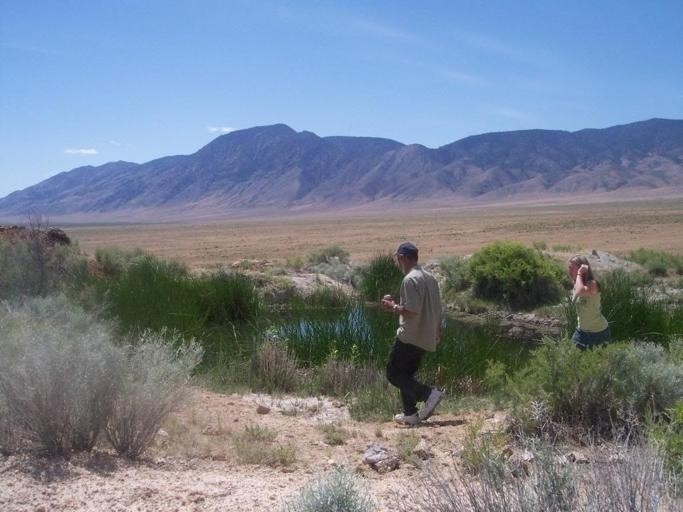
[568,255,610,353]
[378,243,443,422]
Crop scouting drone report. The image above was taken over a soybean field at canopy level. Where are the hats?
[394,242,418,256]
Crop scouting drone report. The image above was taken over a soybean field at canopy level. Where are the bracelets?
[392,304,397,317]
[576,274,583,277]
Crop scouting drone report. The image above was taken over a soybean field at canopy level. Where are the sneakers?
[395,412,421,424]
[422,389,443,420]
